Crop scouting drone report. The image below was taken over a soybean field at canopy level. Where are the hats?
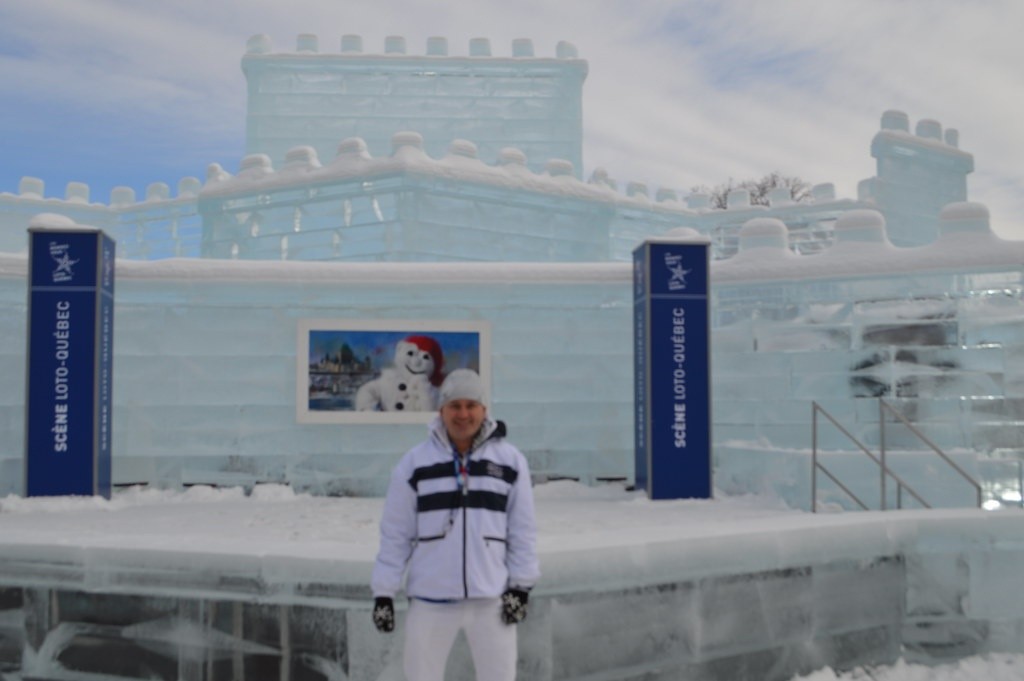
[437,368,487,412]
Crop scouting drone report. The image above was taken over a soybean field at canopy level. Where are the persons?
[370,368,537,681]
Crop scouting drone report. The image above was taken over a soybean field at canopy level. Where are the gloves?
[501,588,529,625]
[372,597,395,633]
